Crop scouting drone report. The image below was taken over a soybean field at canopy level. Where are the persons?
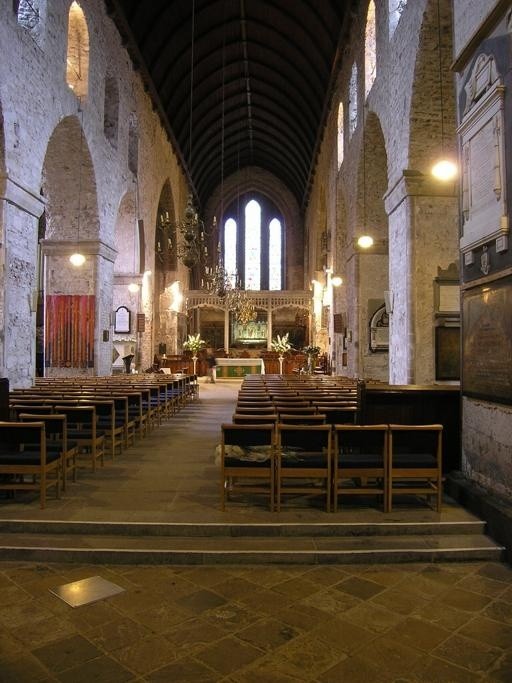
[205,347,217,383]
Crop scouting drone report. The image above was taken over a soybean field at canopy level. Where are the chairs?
[1,372,199,510]
[221,373,467,513]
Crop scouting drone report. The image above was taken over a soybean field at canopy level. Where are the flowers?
[270,331,296,355]
[182,332,205,355]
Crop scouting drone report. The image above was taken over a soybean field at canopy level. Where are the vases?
[192,353,198,375]
[277,354,284,375]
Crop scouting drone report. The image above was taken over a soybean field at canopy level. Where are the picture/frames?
[114,306,131,334]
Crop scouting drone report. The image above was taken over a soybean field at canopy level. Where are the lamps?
[431,0,456,179]
[128,177,141,292]
[331,176,343,286]
[154,0,260,328]
[358,103,374,249]
[69,106,85,266]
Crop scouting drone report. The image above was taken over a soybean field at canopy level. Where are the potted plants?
[302,344,320,371]
